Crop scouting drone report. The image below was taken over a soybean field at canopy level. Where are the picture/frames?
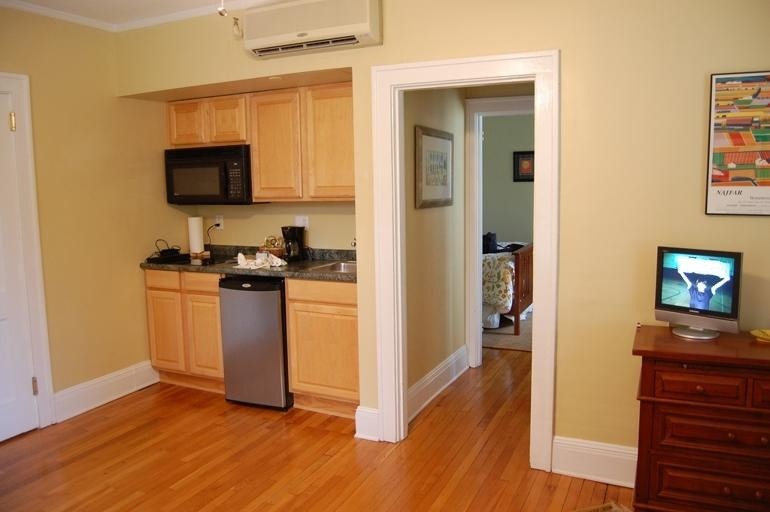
[513,151,533,182]
[703,69,770,216]
[413,124,455,209]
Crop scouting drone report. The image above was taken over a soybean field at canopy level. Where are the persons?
[677,267,730,309]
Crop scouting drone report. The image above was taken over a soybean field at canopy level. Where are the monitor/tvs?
[655,246,743,340]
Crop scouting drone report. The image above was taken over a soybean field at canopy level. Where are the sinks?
[299,259,357,276]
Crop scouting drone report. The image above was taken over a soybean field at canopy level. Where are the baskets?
[259,247,283,257]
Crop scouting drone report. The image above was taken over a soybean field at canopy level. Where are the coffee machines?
[281,224,306,264]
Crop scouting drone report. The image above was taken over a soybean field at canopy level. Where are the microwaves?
[164,144,252,206]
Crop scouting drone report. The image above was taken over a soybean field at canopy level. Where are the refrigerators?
[217,277,292,410]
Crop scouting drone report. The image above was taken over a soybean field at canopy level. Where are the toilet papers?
[190,258,202,266]
[186,214,205,254]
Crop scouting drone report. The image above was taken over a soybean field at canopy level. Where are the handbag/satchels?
[483,232,497,253]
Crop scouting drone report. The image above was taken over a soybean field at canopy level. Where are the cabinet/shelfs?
[630,322,770,511]
[166,94,248,149]
[286,281,360,403]
[144,270,224,381]
[247,83,356,203]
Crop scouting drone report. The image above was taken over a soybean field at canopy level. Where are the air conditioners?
[242,0,384,61]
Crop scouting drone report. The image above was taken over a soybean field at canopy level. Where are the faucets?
[349,235,357,249]
